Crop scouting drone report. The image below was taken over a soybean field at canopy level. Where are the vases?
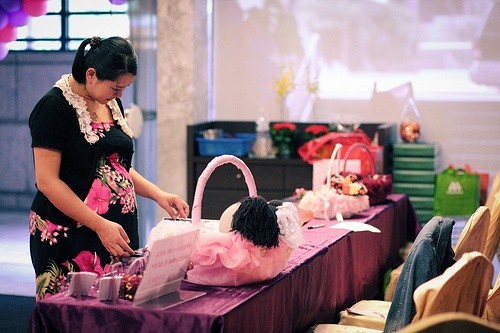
[277,144,291,160]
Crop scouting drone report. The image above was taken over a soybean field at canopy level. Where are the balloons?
[0,0,48,62]
[110,0,129,6]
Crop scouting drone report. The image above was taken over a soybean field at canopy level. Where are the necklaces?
[89,103,99,120]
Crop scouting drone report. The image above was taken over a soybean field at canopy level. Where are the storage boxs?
[196,132,256,157]
[392,140,441,225]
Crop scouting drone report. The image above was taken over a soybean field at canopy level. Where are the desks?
[27,193,424,333]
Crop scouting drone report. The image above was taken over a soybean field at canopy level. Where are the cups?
[68,272,98,296]
[99,277,122,300]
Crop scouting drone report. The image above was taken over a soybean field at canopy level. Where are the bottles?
[399,96,424,145]
[252,117,275,159]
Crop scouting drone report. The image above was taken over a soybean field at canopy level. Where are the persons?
[219,196,286,249]
[28,36,189,301]
[268,200,304,247]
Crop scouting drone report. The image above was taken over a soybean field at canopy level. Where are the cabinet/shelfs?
[185,120,398,221]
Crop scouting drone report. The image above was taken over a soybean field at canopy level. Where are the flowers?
[303,126,329,144]
[270,123,299,148]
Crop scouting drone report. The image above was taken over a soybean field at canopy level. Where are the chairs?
[310,170,500,333]
